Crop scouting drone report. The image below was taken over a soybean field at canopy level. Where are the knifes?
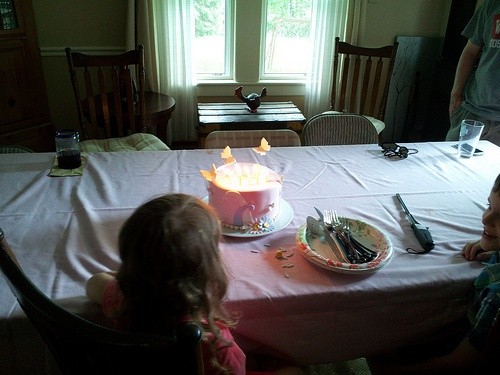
[313,206,324,223]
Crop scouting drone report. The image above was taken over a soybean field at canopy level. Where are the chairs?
[328,37,401,143]
[206,128,301,147]
[65,44,170,151]
[0,228,207,375]
[302,111,377,144]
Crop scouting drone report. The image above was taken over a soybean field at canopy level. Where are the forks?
[323,210,368,263]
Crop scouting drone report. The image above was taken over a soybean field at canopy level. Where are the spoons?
[307,216,352,264]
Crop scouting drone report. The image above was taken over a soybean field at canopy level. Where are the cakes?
[199,136,284,230]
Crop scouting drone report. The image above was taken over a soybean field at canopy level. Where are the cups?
[55,128,82,169]
[457,119,485,160]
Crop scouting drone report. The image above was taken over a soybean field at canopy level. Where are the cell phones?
[456,143,484,155]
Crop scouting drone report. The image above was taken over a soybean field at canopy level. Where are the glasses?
[378,142,409,158]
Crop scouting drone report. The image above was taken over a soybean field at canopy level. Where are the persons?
[367,173,500,375]
[446,0,500,147]
[86,194,304,375]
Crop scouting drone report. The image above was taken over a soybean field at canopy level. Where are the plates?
[296,217,393,273]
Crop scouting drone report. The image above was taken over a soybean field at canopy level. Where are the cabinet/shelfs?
[0,0,56,152]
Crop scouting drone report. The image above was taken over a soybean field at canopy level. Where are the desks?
[0,138,500,375]
[79,90,175,137]
[196,101,305,145]
